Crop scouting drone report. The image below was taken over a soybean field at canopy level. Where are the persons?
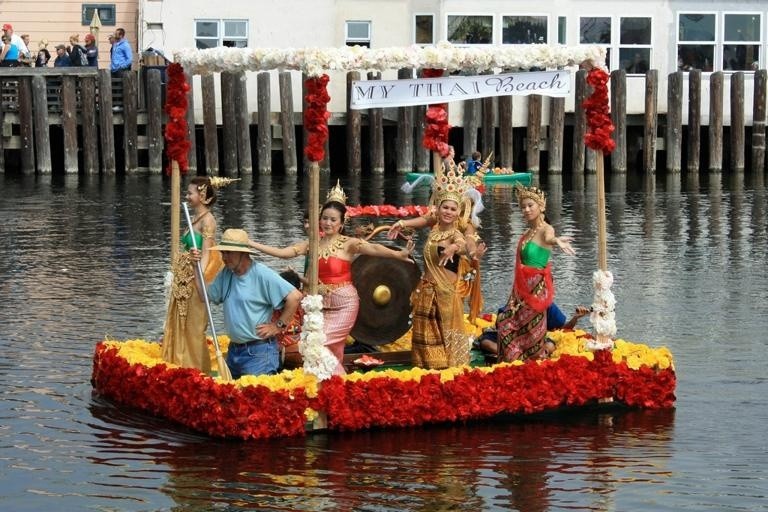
[247,204,415,373]
[443,146,459,177]
[263,272,310,370]
[168,178,218,376]
[37,41,48,68]
[19,34,30,47]
[480,273,592,361]
[627,52,650,75]
[54,45,70,64]
[495,179,575,362]
[388,177,486,329]
[1,23,31,66]
[112,28,132,71]
[0,36,19,67]
[459,156,471,177]
[82,34,99,66]
[411,197,468,371]
[184,230,302,377]
[468,151,485,176]
[67,37,88,65]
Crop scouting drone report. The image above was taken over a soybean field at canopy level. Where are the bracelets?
[399,220,407,228]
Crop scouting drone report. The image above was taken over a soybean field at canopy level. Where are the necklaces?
[521,221,547,251]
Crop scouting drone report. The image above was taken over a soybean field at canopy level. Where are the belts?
[230,336,273,347]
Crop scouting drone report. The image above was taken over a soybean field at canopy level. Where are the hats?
[85,35,95,41]
[207,229,261,256]
[55,44,65,49]
[2,23,12,30]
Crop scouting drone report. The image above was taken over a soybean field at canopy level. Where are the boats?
[90,42,677,440]
[404,170,533,190]
